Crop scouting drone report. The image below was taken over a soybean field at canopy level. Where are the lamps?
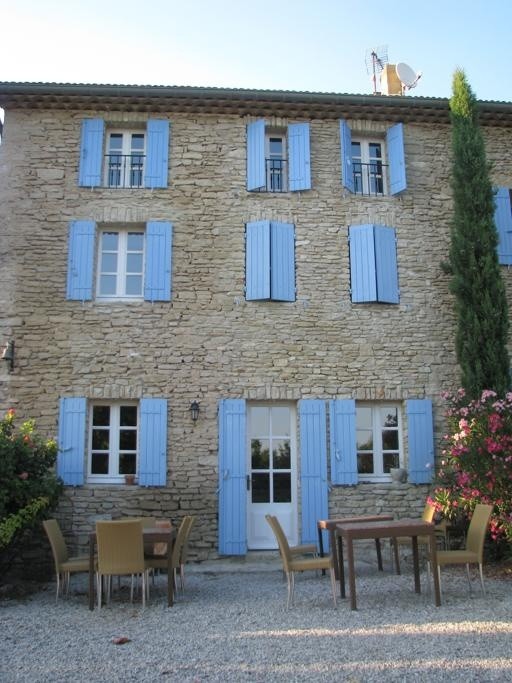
[1,340,16,375]
[188,396,202,425]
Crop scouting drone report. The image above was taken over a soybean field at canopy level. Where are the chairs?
[264,492,493,613]
[41,511,197,614]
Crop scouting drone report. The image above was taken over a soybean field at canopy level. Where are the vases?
[388,465,407,487]
